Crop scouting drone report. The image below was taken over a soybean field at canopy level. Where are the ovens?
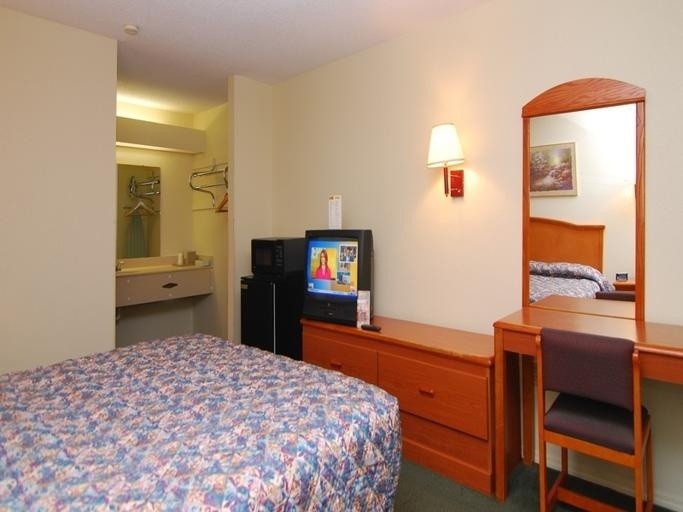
[528,216,615,304]
[0,331,402,512]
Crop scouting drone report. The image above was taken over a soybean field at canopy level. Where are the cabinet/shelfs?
[177,253,184,265]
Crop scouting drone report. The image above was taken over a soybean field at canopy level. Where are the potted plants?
[528,142,578,198]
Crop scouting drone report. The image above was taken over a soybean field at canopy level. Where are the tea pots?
[522,77,646,322]
[117,164,161,259]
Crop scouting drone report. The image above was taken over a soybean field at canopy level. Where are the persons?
[343,247,353,261]
[315,250,331,279]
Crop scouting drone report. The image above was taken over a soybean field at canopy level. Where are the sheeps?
[116,260,125,271]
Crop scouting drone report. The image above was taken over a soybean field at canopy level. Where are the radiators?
[361,323,381,333]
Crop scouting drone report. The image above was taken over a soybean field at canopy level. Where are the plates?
[300,315,522,497]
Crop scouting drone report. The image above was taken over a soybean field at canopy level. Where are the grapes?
[613,281,635,291]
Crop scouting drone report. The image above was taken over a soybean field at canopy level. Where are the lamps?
[595,292,635,302]
[535,326,654,512]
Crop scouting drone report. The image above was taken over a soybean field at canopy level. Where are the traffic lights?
[529,260,602,283]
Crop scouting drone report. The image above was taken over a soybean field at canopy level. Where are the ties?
[299,229,373,326]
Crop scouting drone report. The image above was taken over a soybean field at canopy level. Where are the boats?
[240,276,303,361]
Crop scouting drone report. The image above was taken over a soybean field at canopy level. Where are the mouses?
[123,192,159,218]
[214,192,229,214]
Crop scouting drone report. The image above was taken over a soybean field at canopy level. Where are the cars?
[616,273,628,282]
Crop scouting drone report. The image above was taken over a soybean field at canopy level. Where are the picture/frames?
[530,294,636,319]
[493,307,683,504]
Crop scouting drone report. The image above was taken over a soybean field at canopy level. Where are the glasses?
[426,123,465,198]
